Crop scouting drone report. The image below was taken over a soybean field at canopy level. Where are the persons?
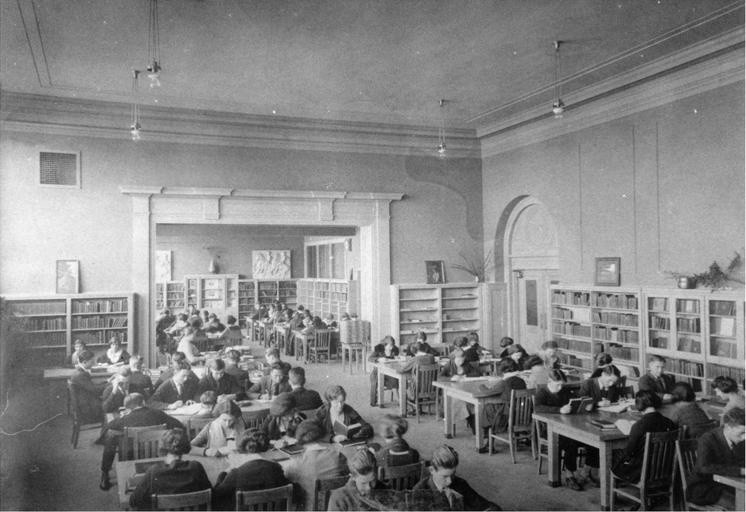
[71,305,502,510]
[368,333,746,512]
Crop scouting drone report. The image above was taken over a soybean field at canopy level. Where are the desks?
[42,368,113,415]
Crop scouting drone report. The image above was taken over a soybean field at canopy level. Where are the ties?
[272,383,280,395]
[178,385,184,396]
[655,377,665,391]
[439,489,451,510]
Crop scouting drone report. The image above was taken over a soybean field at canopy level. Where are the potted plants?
[665,251,744,292]
[450,251,502,283]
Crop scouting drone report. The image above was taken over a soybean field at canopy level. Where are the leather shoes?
[370,397,379,407]
[579,473,600,487]
[100,475,111,490]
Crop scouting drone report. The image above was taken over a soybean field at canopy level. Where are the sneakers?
[562,477,584,492]
[478,441,495,454]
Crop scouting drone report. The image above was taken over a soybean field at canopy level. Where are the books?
[156,277,347,326]
[25,300,128,367]
[552,291,745,402]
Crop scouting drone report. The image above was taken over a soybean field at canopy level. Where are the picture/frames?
[426,260,445,284]
[55,259,79,294]
[595,257,620,287]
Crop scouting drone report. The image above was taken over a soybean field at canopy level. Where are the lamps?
[551,40,565,121]
[148,0,162,85]
[130,70,143,144]
[436,100,447,162]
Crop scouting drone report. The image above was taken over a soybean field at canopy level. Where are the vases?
[209,259,218,273]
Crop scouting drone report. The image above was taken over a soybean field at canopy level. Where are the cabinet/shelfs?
[297,277,356,322]
[643,284,745,403]
[391,282,480,348]
[548,285,644,376]
[185,274,239,326]
[239,279,298,327]
[1,292,136,367]
[157,281,184,310]
